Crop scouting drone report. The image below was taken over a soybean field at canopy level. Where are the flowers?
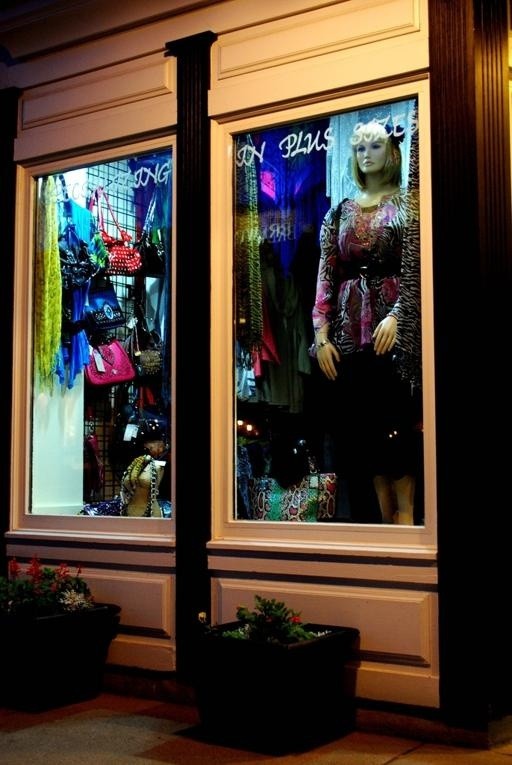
[235,596,313,643]
[2,555,92,617]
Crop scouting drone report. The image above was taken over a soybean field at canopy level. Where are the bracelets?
[314,338,329,352]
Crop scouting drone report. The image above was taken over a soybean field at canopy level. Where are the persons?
[124,455,166,517]
[312,121,418,525]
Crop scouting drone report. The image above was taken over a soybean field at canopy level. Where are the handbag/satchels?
[49,227,128,336]
[95,230,143,278]
[239,439,340,523]
[83,339,137,386]
[83,402,104,494]
[130,227,167,278]
[113,388,167,461]
[128,350,165,380]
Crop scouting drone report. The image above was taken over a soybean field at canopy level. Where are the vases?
[196,617,361,755]
[0,601,122,714]
